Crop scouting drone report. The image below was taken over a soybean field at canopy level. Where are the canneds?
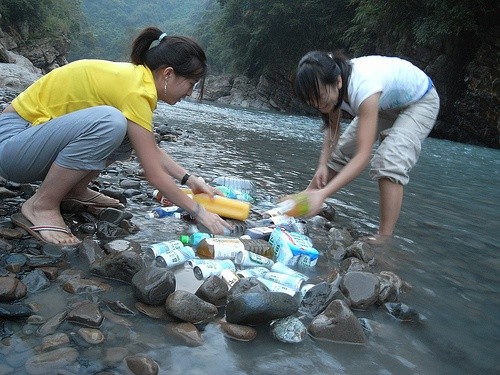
[147,229,319,298]
[262,207,305,234]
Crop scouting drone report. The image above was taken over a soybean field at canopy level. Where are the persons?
[0,27,234,254]
[295,51,440,235]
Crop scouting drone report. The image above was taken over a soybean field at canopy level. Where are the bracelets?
[191,203,200,216]
[181,172,191,185]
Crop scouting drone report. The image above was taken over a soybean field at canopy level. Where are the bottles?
[148,173,327,295]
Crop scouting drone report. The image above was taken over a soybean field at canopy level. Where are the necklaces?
[329,103,341,147]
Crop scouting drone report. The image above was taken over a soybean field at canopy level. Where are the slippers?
[62,193,119,207]
[11,213,80,246]
